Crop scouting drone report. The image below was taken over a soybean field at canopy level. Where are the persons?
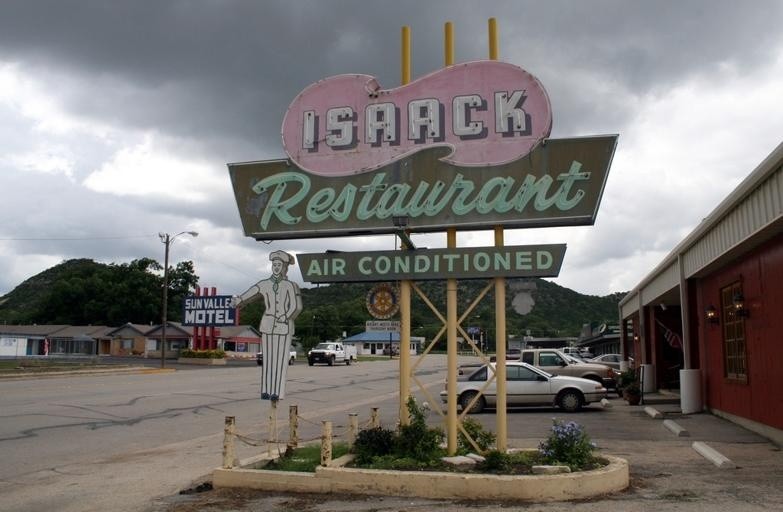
[228,250,302,400]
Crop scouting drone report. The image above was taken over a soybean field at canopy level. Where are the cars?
[489,348,634,372]
[439,361,606,413]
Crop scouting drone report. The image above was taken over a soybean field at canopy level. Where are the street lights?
[157,229,199,369]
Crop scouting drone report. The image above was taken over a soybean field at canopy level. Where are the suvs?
[256,345,296,366]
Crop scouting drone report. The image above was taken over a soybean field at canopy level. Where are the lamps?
[733,290,750,317]
[705,305,719,325]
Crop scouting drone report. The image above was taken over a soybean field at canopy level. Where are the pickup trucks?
[505,347,619,393]
[307,342,357,366]
